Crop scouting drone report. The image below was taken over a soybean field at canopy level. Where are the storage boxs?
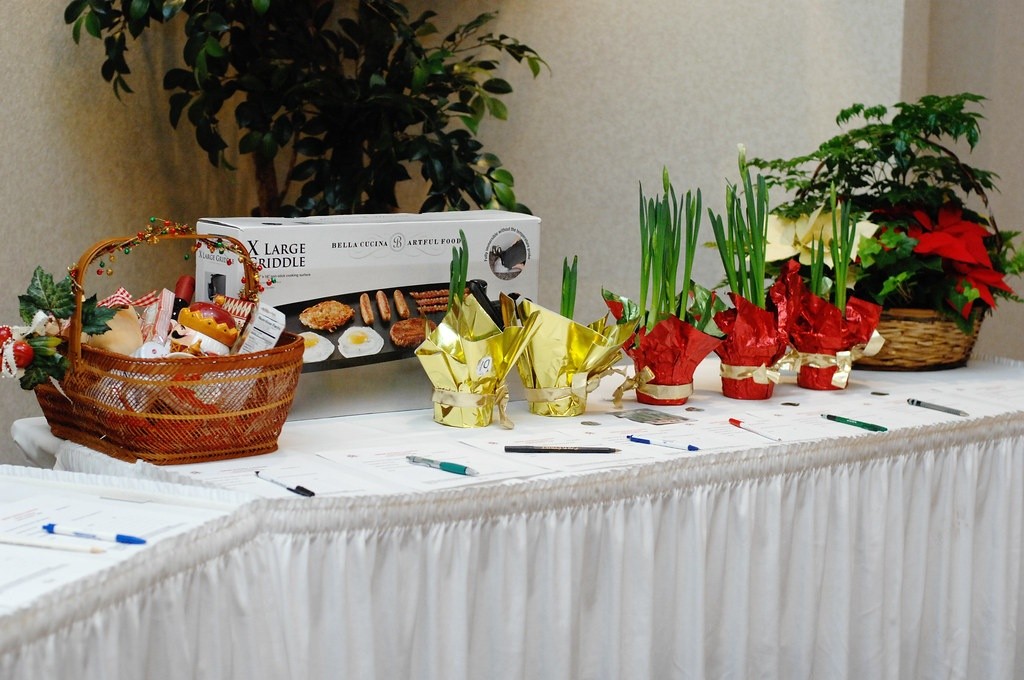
[193,209,544,366]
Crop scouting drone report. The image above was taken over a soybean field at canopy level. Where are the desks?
[0,352,1024,680]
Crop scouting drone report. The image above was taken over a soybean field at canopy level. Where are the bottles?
[171,276,195,325]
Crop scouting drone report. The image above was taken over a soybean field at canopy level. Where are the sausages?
[359,290,410,325]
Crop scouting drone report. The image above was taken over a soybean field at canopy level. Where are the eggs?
[337,326,384,357]
[296,331,335,364]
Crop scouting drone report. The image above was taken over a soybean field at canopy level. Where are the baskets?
[34,232,306,466]
[851,303,985,370]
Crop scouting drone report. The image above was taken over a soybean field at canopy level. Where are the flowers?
[725,88,1023,318]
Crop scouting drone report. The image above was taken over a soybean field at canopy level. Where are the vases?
[862,306,992,367]
